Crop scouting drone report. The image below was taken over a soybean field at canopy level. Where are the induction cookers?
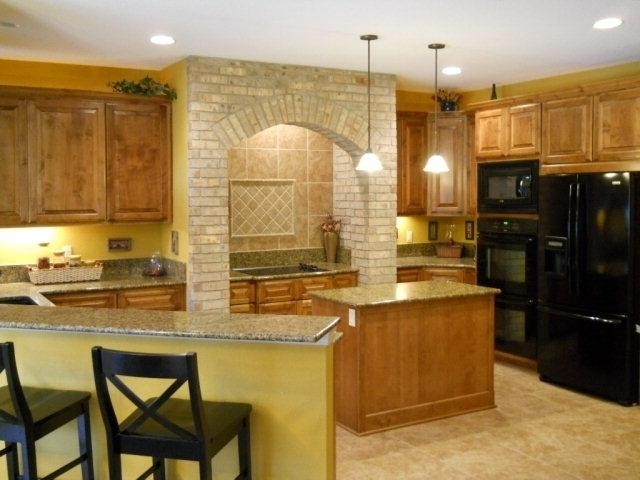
[240,264,329,277]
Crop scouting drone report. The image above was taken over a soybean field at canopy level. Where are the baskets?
[27,264,104,285]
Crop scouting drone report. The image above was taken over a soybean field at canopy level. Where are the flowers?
[446,223,455,240]
[319,214,342,232]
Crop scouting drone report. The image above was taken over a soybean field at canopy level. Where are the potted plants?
[439,88,462,111]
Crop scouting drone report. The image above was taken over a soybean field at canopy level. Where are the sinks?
[0,295,39,305]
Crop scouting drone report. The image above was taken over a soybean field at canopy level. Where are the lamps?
[423,43,449,173]
[356,34,382,171]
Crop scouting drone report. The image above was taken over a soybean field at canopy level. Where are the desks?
[308,279,502,436]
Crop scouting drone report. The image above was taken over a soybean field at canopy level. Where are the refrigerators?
[538,172,638,410]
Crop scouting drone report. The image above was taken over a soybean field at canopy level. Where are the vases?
[448,239,453,246]
[324,231,339,262]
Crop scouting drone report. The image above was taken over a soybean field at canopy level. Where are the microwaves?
[477,159,539,214]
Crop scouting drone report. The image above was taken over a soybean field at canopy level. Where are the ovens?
[475,216,538,368]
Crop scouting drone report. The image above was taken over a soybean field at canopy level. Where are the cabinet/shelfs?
[29,85,173,226]
[539,74,640,176]
[0,85,29,229]
[50,285,186,316]
[396,110,427,216]
[231,272,358,316]
[398,268,476,286]
[427,112,467,219]
[467,93,541,164]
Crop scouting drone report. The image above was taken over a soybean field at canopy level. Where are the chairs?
[0,341,94,480]
[92,345,251,480]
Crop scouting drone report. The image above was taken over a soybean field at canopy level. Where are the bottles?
[148,250,161,273]
[37,242,82,269]
